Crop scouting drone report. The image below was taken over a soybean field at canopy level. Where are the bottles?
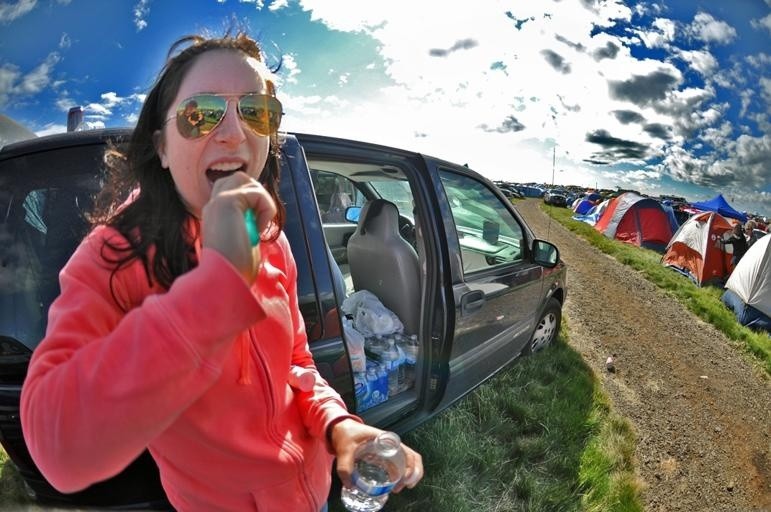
[340,430,409,512]
[73,121,105,131]
[337,333,423,412]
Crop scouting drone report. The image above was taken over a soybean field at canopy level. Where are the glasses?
[160,91,285,140]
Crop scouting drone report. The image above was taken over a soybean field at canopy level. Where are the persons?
[19,30,426,511]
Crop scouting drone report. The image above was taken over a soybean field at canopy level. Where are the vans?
[1,127,569,511]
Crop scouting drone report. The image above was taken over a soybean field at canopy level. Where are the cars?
[444,185,498,232]
[544,189,567,207]
[497,185,525,198]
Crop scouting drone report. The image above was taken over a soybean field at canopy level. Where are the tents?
[513,182,770,330]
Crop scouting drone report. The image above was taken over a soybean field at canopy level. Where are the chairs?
[343,201,420,341]
